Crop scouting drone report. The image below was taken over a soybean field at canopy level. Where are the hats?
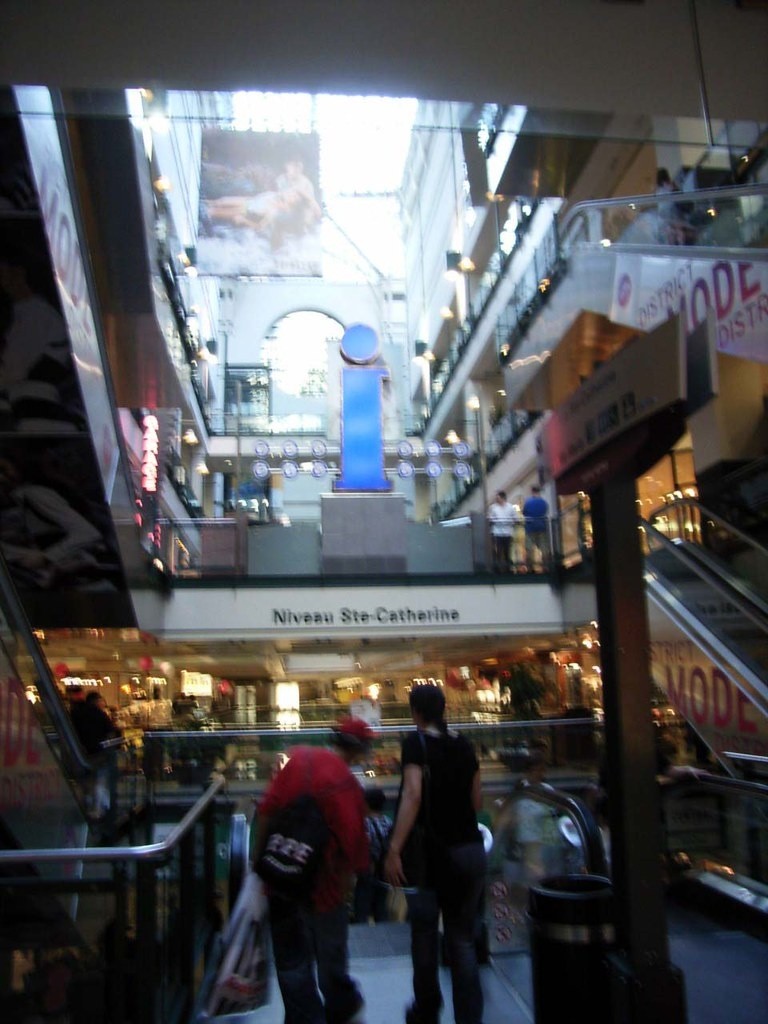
[334,718,373,740]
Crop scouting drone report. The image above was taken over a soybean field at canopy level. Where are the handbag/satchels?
[253,793,330,894]
[195,875,273,1020]
[389,822,432,886]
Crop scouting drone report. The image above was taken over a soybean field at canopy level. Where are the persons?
[251,718,376,1024]
[200,159,322,240]
[0,239,119,592]
[384,685,489,1024]
[653,166,695,245]
[522,485,554,574]
[488,491,521,568]
[510,732,707,883]
[365,788,395,924]
[63,678,123,818]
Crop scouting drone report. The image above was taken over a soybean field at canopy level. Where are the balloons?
[220,678,236,696]
[139,656,153,671]
[55,663,71,679]
